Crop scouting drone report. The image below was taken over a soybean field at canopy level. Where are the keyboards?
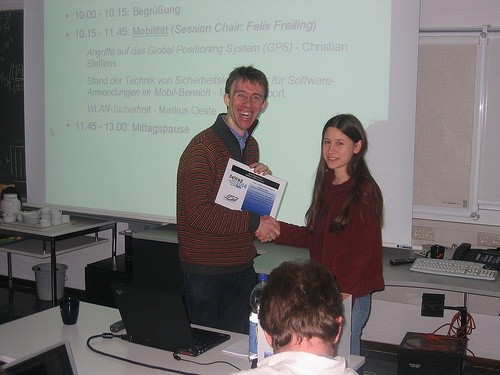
[409,258,497,280]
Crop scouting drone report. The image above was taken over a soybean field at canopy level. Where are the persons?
[237,261,358,375]
[256,115,384,356]
[176,66,281,336]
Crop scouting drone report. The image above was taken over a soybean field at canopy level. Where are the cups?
[62,215,70,223]
[425,246,444,259]
[40,207,62,227]
[59,297,79,325]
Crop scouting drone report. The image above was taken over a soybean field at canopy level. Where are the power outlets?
[490,233,500,247]
[423,227,436,241]
[412,226,423,241]
[477,232,489,246]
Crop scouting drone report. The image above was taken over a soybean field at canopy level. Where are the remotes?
[389,258,415,265]
[109,320,125,333]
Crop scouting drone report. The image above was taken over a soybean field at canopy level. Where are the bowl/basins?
[3,217,16,223]
[22,213,40,224]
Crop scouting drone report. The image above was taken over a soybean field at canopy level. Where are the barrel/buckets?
[32,263,69,300]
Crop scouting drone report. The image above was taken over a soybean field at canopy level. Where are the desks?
[0,300,366,375]
[85,224,500,316]
[0,215,117,307]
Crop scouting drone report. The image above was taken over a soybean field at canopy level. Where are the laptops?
[118,291,231,357]
[0,340,78,375]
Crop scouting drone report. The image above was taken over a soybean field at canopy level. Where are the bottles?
[0,194,21,220]
[248,273,268,368]
